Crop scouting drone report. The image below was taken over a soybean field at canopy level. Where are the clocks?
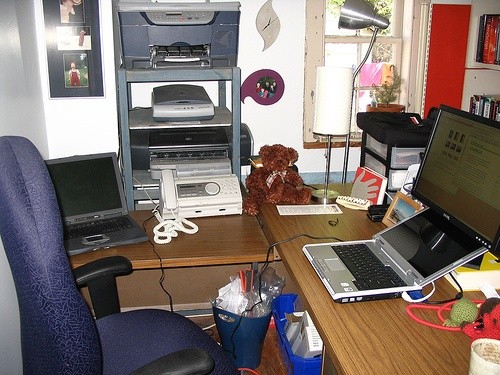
[256,0,280,52]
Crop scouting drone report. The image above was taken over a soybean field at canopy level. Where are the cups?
[467,337,500,375]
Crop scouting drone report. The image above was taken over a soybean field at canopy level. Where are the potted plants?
[366,64,405,112]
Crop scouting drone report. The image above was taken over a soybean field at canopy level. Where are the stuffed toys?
[243,144,312,217]
[460,298,500,348]
[442,298,478,327]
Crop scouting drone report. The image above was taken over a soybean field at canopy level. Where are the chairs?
[0,136,240,375]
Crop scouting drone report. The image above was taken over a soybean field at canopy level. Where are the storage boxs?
[272,289,323,375]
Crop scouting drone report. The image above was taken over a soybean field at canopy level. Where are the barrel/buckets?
[211,301,272,370]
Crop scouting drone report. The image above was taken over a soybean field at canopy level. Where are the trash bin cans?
[211,303,272,368]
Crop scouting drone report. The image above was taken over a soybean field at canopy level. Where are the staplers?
[368,204,390,224]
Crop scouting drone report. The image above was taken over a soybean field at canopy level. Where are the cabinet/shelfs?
[356,112,431,204]
[113,66,242,211]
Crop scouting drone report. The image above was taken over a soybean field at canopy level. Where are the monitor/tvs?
[411,104,500,259]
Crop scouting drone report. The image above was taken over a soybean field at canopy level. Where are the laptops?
[303,206,488,303]
[44,152,150,258]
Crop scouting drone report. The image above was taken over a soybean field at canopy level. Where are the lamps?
[312,64,354,204]
[338,0,389,184]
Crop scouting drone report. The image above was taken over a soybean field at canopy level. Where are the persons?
[60,0,84,22]
[79,29,86,46]
[256,81,277,98]
[69,62,80,86]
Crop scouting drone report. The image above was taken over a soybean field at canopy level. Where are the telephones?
[160,169,244,218]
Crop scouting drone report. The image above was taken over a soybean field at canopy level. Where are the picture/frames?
[382,191,423,227]
[241,69,284,105]
[43,0,104,98]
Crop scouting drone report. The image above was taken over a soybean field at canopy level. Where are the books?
[351,165,388,205]
[476,14,500,65]
[469,95,500,121]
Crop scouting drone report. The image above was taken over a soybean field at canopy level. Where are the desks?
[255,177,500,375]
[70,190,272,319]
